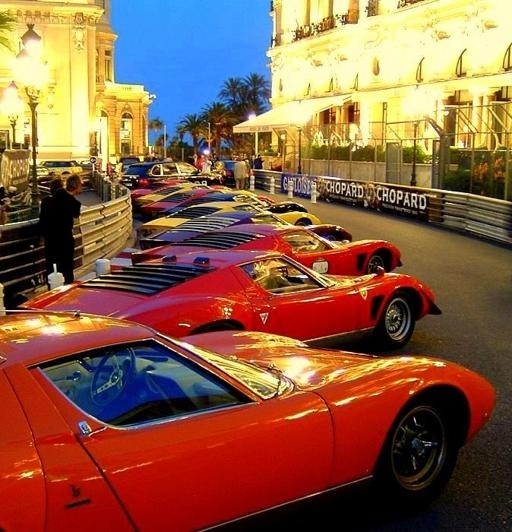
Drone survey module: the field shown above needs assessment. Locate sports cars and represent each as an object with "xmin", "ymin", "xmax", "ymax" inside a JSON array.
[
  {"xmin": 117, "ymin": 155, "xmax": 404, "ymax": 277},
  {"xmin": 0, "ymin": 309, "xmax": 499, "ymax": 530},
  {"xmin": 16, "ymin": 248, "xmax": 442, "ymax": 347}
]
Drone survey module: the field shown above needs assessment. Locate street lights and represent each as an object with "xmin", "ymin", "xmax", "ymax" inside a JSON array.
[
  {"xmin": 400, "ymin": 88, "xmax": 428, "ymax": 188},
  {"xmin": 1, "ymin": 81, "xmax": 24, "ymax": 146},
  {"xmin": 12, "ymin": 20, "xmax": 46, "ymax": 220},
  {"xmin": 287, "ymin": 106, "xmax": 312, "ymax": 174}
]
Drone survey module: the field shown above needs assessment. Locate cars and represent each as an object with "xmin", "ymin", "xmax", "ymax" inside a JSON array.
[{"xmin": 29, "ymin": 159, "xmax": 85, "ymax": 177}]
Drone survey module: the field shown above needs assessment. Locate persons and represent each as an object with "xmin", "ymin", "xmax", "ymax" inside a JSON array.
[
  {"xmin": 196, "ymin": 153, "xmax": 217, "ymax": 175},
  {"xmin": 0, "ymin": 187, "xmax": 10, "ymax": 223},
  {"xmin": 145, "ymin": 152, "xmax": 159, "ymax": 162},
  {"xmin": 254, "ymin": 154, "xmax": 265, "ymax": 169},
  {"xmin": 39, "ymin": 174, "xmax": 84, "ymax": 291},
  {"xmin": 235, "ymin": 159, "xmax": 251, "ymax": 189}
]
